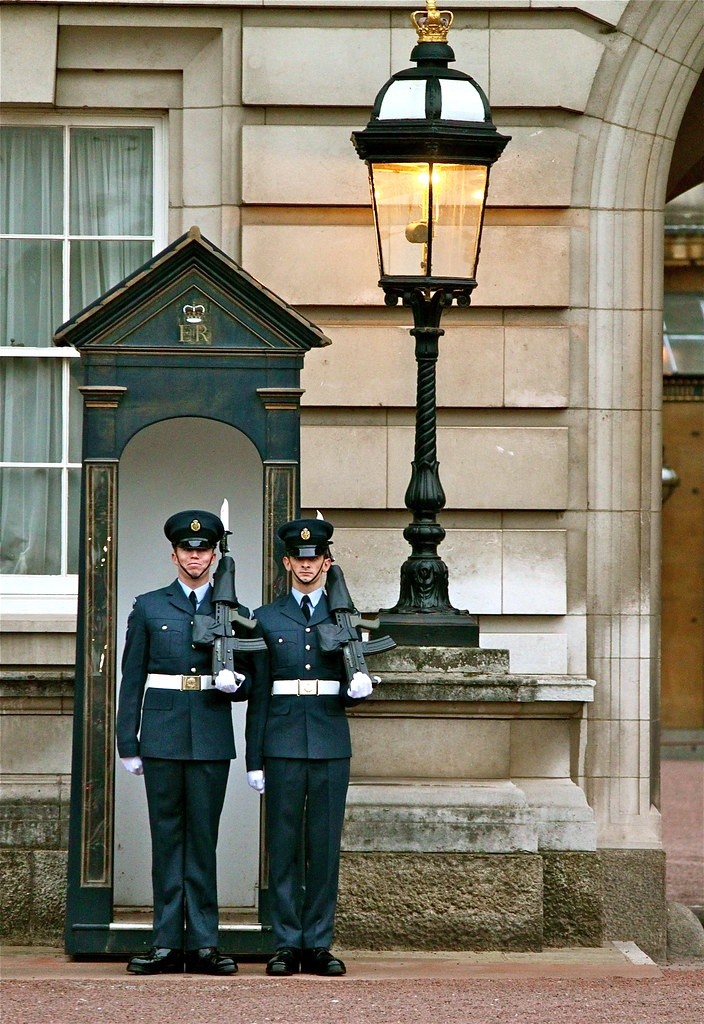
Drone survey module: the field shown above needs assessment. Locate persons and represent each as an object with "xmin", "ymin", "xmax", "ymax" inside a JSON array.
[
  {"xmin": 245, "ymin": 519, "xmax": 375, "ymax": 975},
  {"xmin": 117, "ymin": 505, "xmax": 252, "ymax": 974}
]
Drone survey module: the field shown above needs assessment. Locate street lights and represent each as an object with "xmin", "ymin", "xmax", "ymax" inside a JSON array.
[{"xmin": 356, "ymin": 0, "xmax": 516, "ymax": 651}]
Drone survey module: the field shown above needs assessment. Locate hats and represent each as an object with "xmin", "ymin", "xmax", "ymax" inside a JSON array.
[
  {"xmin": 164, "ymin": 510, "xmax": 225, "ymax": 549},
  {"xmin": 277, "ymin": 519, "xmax": 334, "ymax": 557}
]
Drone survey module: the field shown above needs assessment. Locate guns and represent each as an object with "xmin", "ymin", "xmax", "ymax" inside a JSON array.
[
  {"xmin": 315, "ymin": 509, "xmax": 397, "ymax": 689},
  {"xmin": 211, "ymin": 497, "xmax": 269, "ymax": 685}
]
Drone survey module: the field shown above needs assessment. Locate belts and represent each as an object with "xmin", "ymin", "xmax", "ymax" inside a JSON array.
[
  {"xmin": 271, "ymin": 679, "xmax": 340, "ymax": 696},
  {"xmin": 147, "ymin": 674, "xmax": 216, "ymax": 691}
]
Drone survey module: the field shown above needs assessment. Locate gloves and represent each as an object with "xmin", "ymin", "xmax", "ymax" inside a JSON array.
[
  {"xmin": 347, "ymin": 672, "xmax": 373, "ymax": 699},
  {"xmin": 215, "ymin": 669, "xmax": 245, "ymax": 693},
  {"xmin": 120, "ymin": 756, "xmax": 143, "ymax": 775},
  {"xmin": 247, "ymin": 769, "xmax": 264, "ymax": 793}
]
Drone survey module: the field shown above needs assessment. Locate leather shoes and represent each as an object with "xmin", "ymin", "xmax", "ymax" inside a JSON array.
[
  {"xmin": 185, "ymin": 947, "xmax": 238, "ymax": 974},
  {"xmin": 301, "ymin": 946, "xmax": 346, "ymax": 975},
  {"xmin": 127, "ymin": 946, "xmax": 184, "ymax": 974},
  {"xmin": 266, "ymin": 947, "xmax": 299, "ymax": 975}
]
